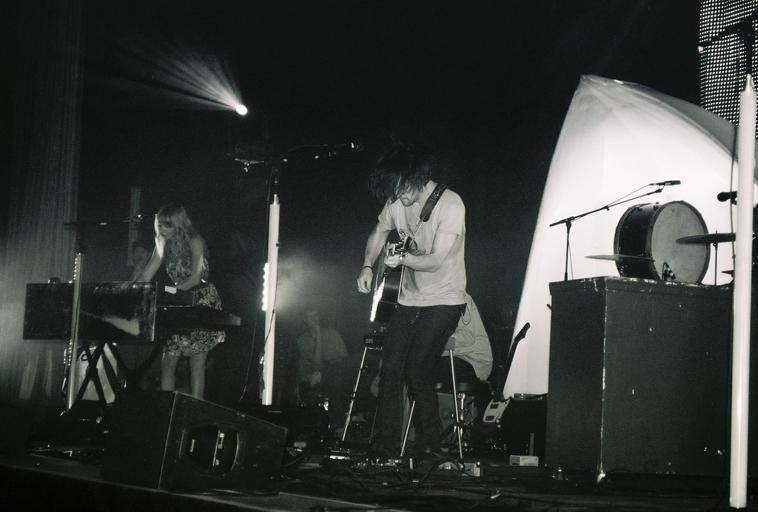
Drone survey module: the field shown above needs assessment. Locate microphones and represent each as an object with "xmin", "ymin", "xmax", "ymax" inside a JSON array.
[
  {"xmin": 346, "ymin": 138, "xmax": 366, "ymax": 152},
  {"xmin": 653, "ymin": 180, "xmax": 681, "ymax": 185},
  {"xmin": 110, "ymin": 213, "xmax": 144, "ymax": 225},
  {"xmin": 717, "ymin": 191, "xmax": 736, "ymax": 202}
]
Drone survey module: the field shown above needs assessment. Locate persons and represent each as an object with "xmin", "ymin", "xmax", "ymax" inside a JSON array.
[
  {"xmin": 123, "ymin": 240, "xmax": 149, "ymax": 282},
  {"xmin": 353, "ymin": 137, "xmax": 469, "ymax": 463},
  {"xmin": 430, "ymin": 289, "xmax": 493, "ymax": 389},
  {"xmin": 294, "ymin": 300, "xmax": 353, "ymax": 447},
  {"xmin": 131, "ymin": 196, "xmax": 225, "ymax": 400}
]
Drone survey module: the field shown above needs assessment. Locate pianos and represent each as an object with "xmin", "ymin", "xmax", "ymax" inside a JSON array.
[{"xmin": 22, "ymin": 281, "xmax": 241, "ymax": 343}]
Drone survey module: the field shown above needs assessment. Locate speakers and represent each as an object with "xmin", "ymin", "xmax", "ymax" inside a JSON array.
[
  {"xmin": 545, "ymin": 275, "xmax": 732, "ymax": 485},
  {"xmin": 103, "ymin": 387, "xmax": 288, "ymax": 492}
]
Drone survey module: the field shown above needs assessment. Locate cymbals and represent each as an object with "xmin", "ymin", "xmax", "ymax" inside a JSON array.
[
  {"xmin": 674, "ymin": 233, "xmax": 735, "ymax": 244},
  {"xmin": 585, "ymin": 255, "xmax": 656, "ymax": 264}
]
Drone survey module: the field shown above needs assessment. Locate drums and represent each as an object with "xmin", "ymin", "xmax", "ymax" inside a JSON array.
[{"xmin": 614, "ymin": 202, "xmax": 710, "ymax": 284}]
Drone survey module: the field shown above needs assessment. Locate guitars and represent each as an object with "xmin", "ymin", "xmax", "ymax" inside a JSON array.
[
  {"xmin": 369, "ymin": 234, "xmax": 414, "ymax": 322},
  {"xmin": 483, "ymin": 323, "xmax": 530, "ymax": 453}
]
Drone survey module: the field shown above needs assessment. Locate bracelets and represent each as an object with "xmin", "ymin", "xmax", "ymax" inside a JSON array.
[{"xmin": 360, "ymin": 265, "xmax": 374, "ymax": 271}]
